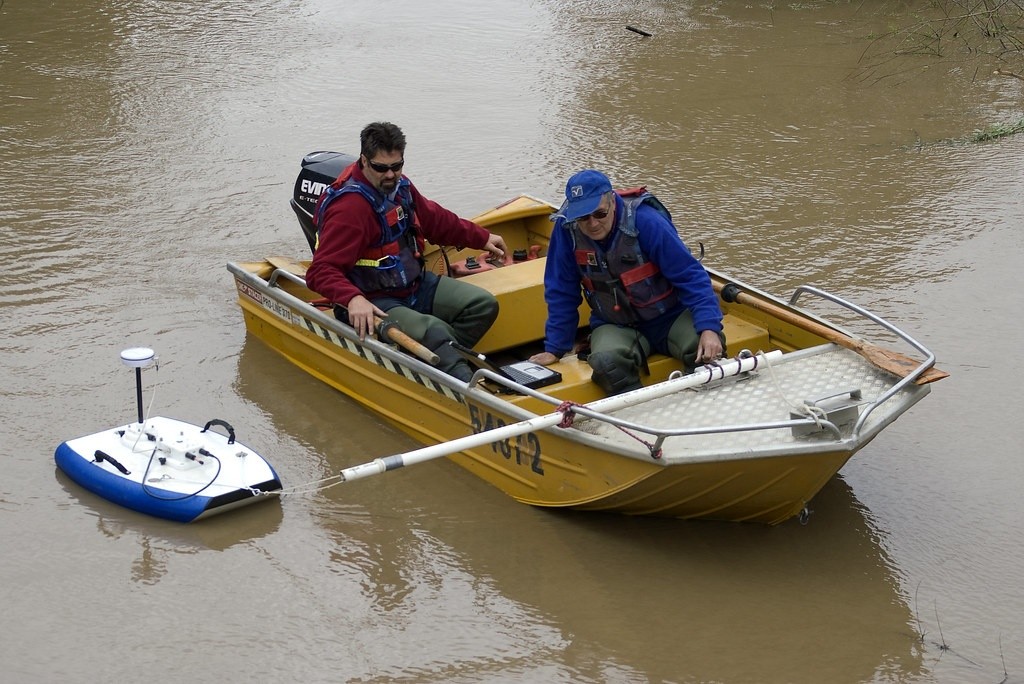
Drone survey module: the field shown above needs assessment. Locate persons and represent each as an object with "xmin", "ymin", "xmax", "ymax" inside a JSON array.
[
  {"xmin": 529, "ymin": 169, "xmax": 729, "ymax": 397},
  {"xmin": 305, "ymin": 122, "xmax": 507, "ymax": 382}
]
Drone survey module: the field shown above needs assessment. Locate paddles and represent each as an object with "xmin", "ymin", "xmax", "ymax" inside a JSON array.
[
  {"xmin": 266, "ymin": 255, "xmax": 441, "ymax": 366},
  {"xmin": 712, "ymin": 277, "xmax": 950, "ymax": 386}
]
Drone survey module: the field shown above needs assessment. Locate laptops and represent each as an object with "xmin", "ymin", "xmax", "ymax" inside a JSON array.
[{"xmin": 448, "ymin": 341, "xmax": 562, "ymax": 395}]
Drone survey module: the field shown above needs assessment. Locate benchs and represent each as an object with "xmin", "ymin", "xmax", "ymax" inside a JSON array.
[
  {"xmin": 467, "ymin": 313, "xmax": 771, "ymax": 416},
  {"xmin": 321, "ymin": 256, "xmax": 593, "ymax": 354}
]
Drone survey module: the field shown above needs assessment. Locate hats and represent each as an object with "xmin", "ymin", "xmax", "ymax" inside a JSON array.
[{"xmin": 566, "ymin": 169, "xmax": 612, "ymax": 221}]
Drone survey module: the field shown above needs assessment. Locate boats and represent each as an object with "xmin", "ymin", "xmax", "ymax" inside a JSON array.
[{"xmin": 222, "ymin": 194, "xmax": 952, "ymax": 525}]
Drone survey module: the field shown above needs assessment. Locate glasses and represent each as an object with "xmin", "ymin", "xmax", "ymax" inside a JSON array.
[
  {"xmin": 367, "ymin": 156, "xmax": 404, "ymax": 173},
  {"xmin": 575, "ymin": 199, "xmax": 610, "ymax": 222}
]
